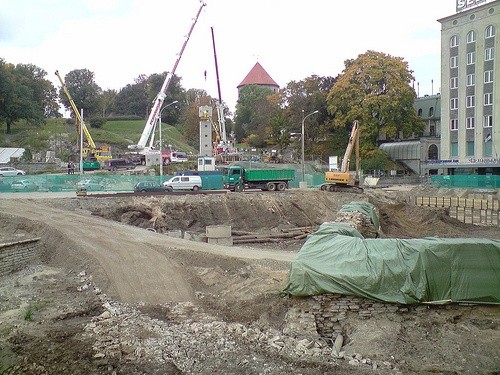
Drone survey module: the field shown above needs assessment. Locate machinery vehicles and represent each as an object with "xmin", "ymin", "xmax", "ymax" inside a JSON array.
[
  {"xmin": 320, "ymin": 117, "xmax": 380, "ymax": 194},
  {"xmin": 117, "ymin": 0, "xmax": 291, "ymax": 166},
  {"xmin": 54, "ymin": 70, "xmax": 112, "ymax": 171}
]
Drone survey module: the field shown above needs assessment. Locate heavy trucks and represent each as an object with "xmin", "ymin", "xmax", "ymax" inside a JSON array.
[{"xmin": 221, "ymin": 165, "xmax": 296, "ymax": 193}]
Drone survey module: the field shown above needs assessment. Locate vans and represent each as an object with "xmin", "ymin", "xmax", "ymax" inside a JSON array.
[{"xmin": 162, "ymin": 175, "xmax": 202, "ymax": 191}]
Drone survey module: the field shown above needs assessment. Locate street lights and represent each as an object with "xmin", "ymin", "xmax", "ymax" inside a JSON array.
[
  {"xmin": 302, "ymin": 109, "xmax": 319, "ymax": 182},
  {"xmin": 159, "ymin": 100, "xmax": 179, "ymax": 177}
]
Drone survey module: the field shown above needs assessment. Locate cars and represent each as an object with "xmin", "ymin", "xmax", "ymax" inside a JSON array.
[
  {"xmin": 0, "ymin": 166, "xmax": 26, "ymax": 177},
  {"xmin": 105, "ymin": 157, "xmax": 137, "ymax": 172},
  {"xmin": 83, "ymin": 156, "xmax": 102, "ymax": 171},
  {"xmin": 133, "ymin": 180, "xmax": 174, "ymax": 193}
]
